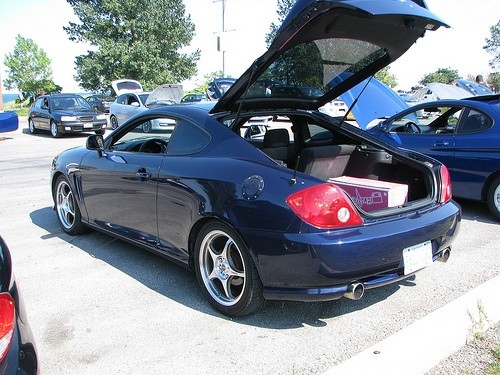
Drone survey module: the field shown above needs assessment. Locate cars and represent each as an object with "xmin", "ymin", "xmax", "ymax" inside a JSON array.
[
  {"xmin": 424, "ymin": 78, "xmax": 496, "ymax": 124},
  {"xmin": 109, "ymin": 79, "xmax": 184, "ymax": 133},
  {"xmin": 27, "ymin": 92, "xmax": 108, "ymax": 138},
  {"xmin": 50, "ymin": 0, "xmax": 465, "ymax": 319},
  {"xmin": 85, "ymin": 94, "xmax": 115, "ymax": 113},
  {"xmin": 329, "ymin": 73, "xmax": 500, "ymax": 222},
  {"xmin": 397, "ymin": 93, "xmax": 429, "ymax": 118},
  {"xmin": 1, "ymin": 235, "xmax": 39, "ymax": 375},
  {"xmin": 181, "ymin": 93, "xmax": 207, "ymax": 102}
]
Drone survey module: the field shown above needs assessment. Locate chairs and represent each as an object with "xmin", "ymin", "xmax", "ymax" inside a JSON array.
[
  {"xmin": 463, "ymin": 114, "xmax": 482, "ymax": 130},
  {"xmin": 262, "ymin": 129, "xmax": 296, "ymax": 169}
]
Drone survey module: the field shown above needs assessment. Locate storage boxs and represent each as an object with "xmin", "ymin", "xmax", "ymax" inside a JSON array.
[{"xmin": 326, "ymin": 176, "xmax": 408, "ymax": 212}]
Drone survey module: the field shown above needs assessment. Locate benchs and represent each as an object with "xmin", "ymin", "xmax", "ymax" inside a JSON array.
[{"xmin": 296, "ymin": 144, "xmax": 366, "ymax": 182}]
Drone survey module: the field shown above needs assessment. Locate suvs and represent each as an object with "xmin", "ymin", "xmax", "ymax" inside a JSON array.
[{"xmin": 268, "ymin": 84, "xmax": 350, "ymax": 120}]
